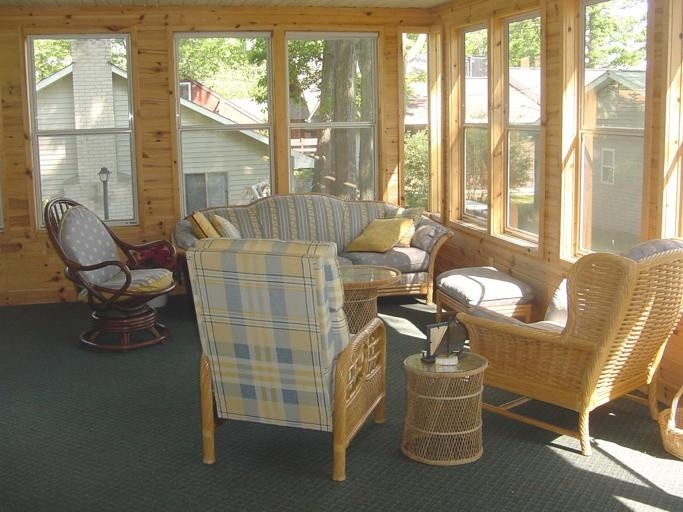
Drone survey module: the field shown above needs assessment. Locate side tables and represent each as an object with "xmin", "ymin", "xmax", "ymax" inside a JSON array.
[{"xmin": 398, "ymin": 350, "xmax": 490, "ymax": 468}]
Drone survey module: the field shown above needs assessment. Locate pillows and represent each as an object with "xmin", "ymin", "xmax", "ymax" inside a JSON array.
[
  {"xmin": 382, "ymin": 205, "xmax": 423, "ymax": 248},
  {"xmin": 187, "ymin": 209, "xmax": 219, "ymax": 241},
  {"xmin": 211, "ymin": 212, "xmax": 244, "ymax": 241},
  {"xmin": 342, "ymin": 215, "xmax": 407, "ymax": 253}
]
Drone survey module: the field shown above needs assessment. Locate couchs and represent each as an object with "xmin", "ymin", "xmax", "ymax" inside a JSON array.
[
  {"xmin": 170, "ymin": 194, "xmax": 455, "ymax": 308},
  {"xmin": 185, "ymin": 234, "xmax": 388, "ymax": 482},
  {"xmin": 454, "ymin": 239, "xmax": 683, "ymax": 457}
]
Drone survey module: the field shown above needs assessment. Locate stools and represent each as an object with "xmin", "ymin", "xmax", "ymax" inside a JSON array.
[{"xmin": 434, "ymin": 265, "xmax": 534, "ymax": 340}]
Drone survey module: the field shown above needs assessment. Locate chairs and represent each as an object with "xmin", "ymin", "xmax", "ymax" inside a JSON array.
[{"xmin": 42, "ymin": 194, "xmax": 180, "ymax": 353}]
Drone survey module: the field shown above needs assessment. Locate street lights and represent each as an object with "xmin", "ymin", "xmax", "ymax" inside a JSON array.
[{"xmin": 97, "ymin": 166, "xmax": 112, "ymax": 221}]
